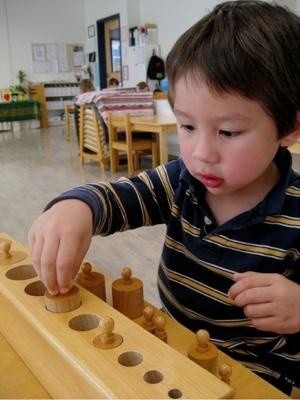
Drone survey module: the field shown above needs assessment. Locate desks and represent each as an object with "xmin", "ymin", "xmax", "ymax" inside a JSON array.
[
  {"xmin": 0, "ymin": 101, "xmax": 41, "ymax": 131},
  {"xmin": 130, "ymin": 116, "xmax": 177, "ymax": 165},
  {"xmin": 76, "ymin": 92, "xmax": 154, "ymax": 172}
]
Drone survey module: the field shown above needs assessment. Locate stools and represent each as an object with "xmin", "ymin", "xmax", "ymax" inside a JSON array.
[
  {"xmin": 0, "ymin": 117, "xmax": 12, "ymax": 132},
  {"xmin": 65, "ymin": 104, "xmax": 77, "ymax": 141}
]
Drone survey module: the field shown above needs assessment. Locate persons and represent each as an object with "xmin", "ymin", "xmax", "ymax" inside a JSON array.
[
  {"xmin": 109, "ymin": 77, "xmax": 120, "ymax": 88},
  {"xmin": 26, "ymin": 0, "xmax": 300, "ymax": 397},
  {"xmin": 77, "ymin": 79, "xmax": 98, "ymax": 105}
]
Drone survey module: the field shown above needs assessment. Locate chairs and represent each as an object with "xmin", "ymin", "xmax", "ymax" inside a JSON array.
[{"xmin": 108, "ymin": 114, "xmax": 158, "ymax": 178}]
